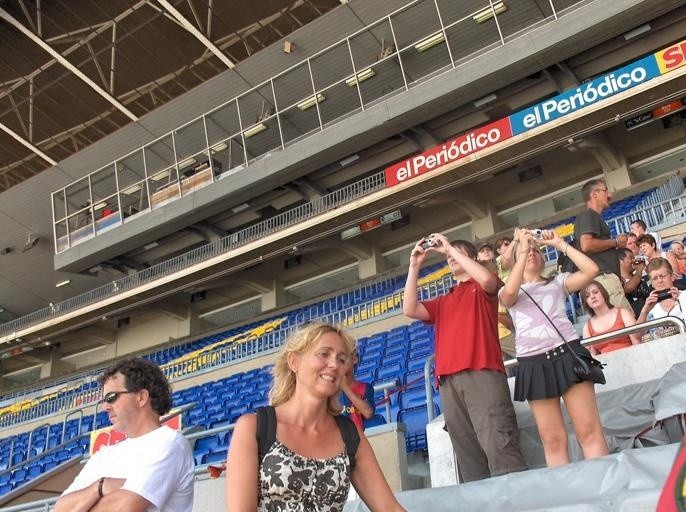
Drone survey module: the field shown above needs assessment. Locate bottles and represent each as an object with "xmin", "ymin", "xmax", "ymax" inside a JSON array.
[{"xmin": 646, "ymin": 313, "xmax": 661, "ymax": 340}]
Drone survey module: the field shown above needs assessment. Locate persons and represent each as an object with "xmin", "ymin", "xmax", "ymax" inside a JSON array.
[
  {"xmin": 403, "ymin": 233, "xmax": 527, "ymax": 484},
  {"xmin": 574, "ymin": 179, "xmax": 637, "ymax": 317},
  {"xmin": 225, "ymin": 321, "xmax": 408, "ymax": 512},
  {"xmin": 51, "ymin": 358, "xmax": 196, "ymax": 511},
  {"xmin": 580, "ymin": 219, "xmax": 686, "ymax": 355},
  {"xmin": 336, "ymin": 348, "xmax": 377, "ymax": 432},
  {"xmin": 497, "ymin": 228, "xmax": 609, "ymax": 467},
  {"xmin": 474, "ymin": 236, "xmax": 518, "ymax": 359}
]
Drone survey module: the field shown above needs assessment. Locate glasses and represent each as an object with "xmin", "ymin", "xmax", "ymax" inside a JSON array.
[{"xmin": 102, "ymin": 389, "xmax": 138, "ymax": 403}]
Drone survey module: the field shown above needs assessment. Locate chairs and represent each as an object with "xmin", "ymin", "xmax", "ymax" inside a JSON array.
[{"xmin": 0, "ymin": 184, "xmax": 662, "ymax": 510}]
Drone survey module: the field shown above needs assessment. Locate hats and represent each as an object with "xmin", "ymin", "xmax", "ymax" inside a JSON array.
[{"xmin": 476, "ymin": 241, "xmax": 493, "ymax": 252}]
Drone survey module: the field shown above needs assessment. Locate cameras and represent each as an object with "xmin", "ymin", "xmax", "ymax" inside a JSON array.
[
  {"xmin": 633, "ymin": 256, "xmax": 647, "ymax": 265},
  {"xmin": 420, "ymin": 235, "xmax": 438, "ymax": 249},
  {"xmin": 653, "ymin": 288, "xmax": 672, "ymax": 302},
  {"xmin": 529, "ymin": 228, "xmax": 545, "ymax": 240}
]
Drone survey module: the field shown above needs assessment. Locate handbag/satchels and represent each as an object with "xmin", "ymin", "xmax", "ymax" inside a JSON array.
[
  {"xmin": 557, "ymin": 240, "xmax": 581, "ymax": 272},
  {"xmin": 574, "ymin": 351, "xmax": 605, "ymax": 384},
  {"xmin": 641, "ymin": 328, "xmax": 676, "ymax": 343}
]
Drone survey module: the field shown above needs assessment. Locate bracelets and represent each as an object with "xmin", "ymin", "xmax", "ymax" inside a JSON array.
[{"xmin": 99, "ymin": 477, "xmax": 105, "ymax": 500}]
